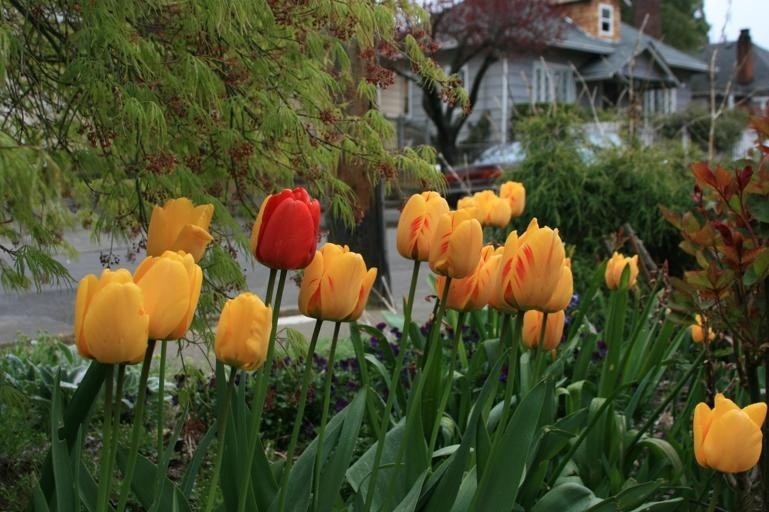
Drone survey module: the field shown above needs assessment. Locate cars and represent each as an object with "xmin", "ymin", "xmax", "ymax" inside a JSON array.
[{"xmin": 444, "ymin": 140, "xmax": 525, "ymax": 209}]
